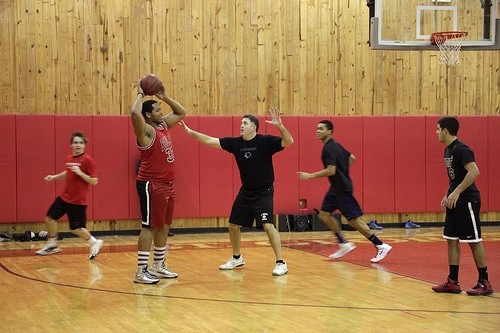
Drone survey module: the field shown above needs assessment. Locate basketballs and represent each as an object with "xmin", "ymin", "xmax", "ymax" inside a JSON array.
[{"xmin": 140, "ymin": 74, "xmax": 163, "ymax": 95}]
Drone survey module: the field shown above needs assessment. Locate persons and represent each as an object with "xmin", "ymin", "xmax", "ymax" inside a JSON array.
[
  {"xmin": 296, "ymin": 120, "xmax": 392, "ymax": 263},
  {"xmin": 431, "ymin": 117, "xmax": 494, "ymax": 296},
  {"xmin": 131, "ymin": 78, "xmax": 186, "ymax": 283},
  {"xmin": 36, "ymin": 131, "xmax": 104, "ymax": 260},
  {"xmin": 177, "ymin": 107, "xmax": 294, "ymax": 275}
]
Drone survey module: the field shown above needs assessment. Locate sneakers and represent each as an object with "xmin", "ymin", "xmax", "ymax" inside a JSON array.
[
  {"xmin": 148, "ymin": 261, "xmax": 179, "ymax": 278},
  {"xmin": 432, "ymin": 276, "xmax": 462, "ymax": 293},
  {"xmin": 219, "ymin": 254, "xmax": 245, "ymax": 270},
  {"xmin": 466, "ymin": 280, "xmax": 494, "ymax": 296},
  {"xmin": 36, "ymin": 242, "xmax": 61, "ymax": 255},
  {"xmin": 369, "ymin": 220, "xmax": 383, "ymax": 230},
  {"xmin": 89, "ymin": 239, "xmax": 104, "ymax": 259},
  {"xmin": 328, "ymin": 241, "xmax": 357, "ymax": 260},
  {"xmin": 370, "ymin": 243, "xmax": 392, "ymax": 263},
  {"xmin": 405, "ymin": 220, "xmax": 421, "ymax": 229},
  {"xmin": 134, "ymin": 265, "xmax": 160, "ymax": 284},
  {"xmin": 272, "ymin": 260, "xmax": 288, "ymax": 276}
]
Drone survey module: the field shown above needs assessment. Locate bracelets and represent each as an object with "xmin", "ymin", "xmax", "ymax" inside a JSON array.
[{"xmin": 137, "ymin": 92, "xmax": 143, "ymax": 98}]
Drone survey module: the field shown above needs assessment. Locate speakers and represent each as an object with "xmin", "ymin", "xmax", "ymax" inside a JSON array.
[
  {"xmin": 277, "ymin": 214, "xmax": 311, "ymax": 233},
  {"xmin": 308, "ymin": 213, "xmax": 343, "ymax": 231}
]
